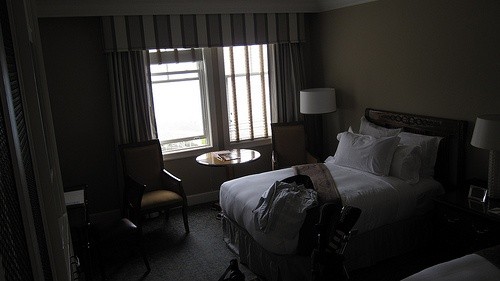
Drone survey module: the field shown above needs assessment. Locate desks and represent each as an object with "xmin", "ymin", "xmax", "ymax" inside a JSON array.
[
  {"xmin": 62, "ymin": 176, "xmax": 93, "ymax": 279},
  {"xmin": 196, "ymin": 148, "xmax": 261, "ymax": 211}
]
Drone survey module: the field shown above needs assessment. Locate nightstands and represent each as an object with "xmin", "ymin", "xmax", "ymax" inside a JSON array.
[{"xmin": 439, "ymin": 190, "xmax": 500, "ymax": 253}]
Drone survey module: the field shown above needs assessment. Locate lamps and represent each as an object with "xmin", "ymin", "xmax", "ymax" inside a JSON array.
[
  {"xmin": 299, "ymin": 88, "xmax": 336, "ymax": 162},
  {"xmin": 471, "ymin": 115, "xmax": 500, "ymax": 199}
]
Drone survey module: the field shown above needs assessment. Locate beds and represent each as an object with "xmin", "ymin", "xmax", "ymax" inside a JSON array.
[
  {"xmin": 400, "ymin": 244, "xmax": 500, "ymax": 281},
  {"xmin": 217, "ymin": 108, "xmax": 467, "ymax": 281}
]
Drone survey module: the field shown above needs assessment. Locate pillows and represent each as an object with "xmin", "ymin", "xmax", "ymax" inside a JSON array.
[{"xmin": 324, "ymin": 115, "xmax": 445, "ymax": 181}]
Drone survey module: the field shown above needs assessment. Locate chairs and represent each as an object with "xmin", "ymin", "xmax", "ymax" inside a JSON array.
[
  {"xmin": 86, "ymin": 175, "xmax": 152, "ymax": 281},
  {"xmin": 270, "ymin": 122, "xmax": 318, "ymax": 170},
  {"xmin": 117, "ymin": 140, "xmax": 190, "ymax": 237}
]
